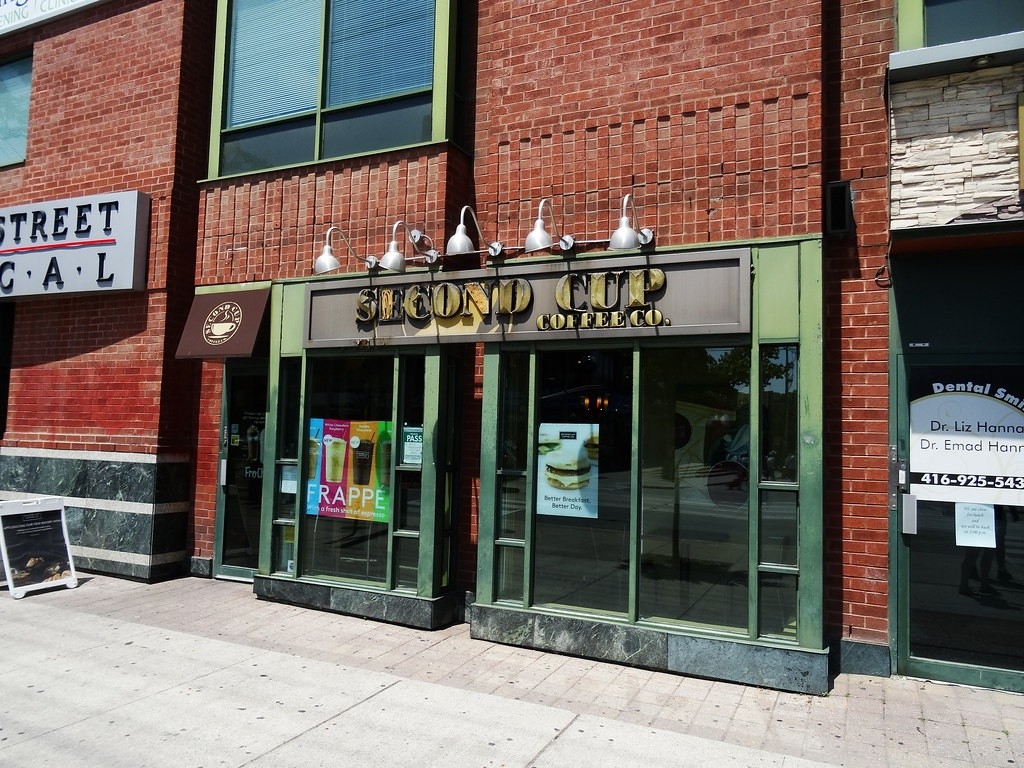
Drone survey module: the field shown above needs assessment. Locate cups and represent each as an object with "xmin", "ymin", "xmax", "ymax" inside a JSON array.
[
  {"xmin": 326, "ymin": 442, "xmax": 346, "ymax": 482},
  {"xmin": 353, "ymin": 443, "xmax": 375, "ymax": 485},
  {"xmin": 380, "ymin": 444, "xmax": 391, "ymax": 485},
  {"xmin": 247, "ymin": 434, "xmax": 258, "ymax": 461},
  {"xmin": 260, "ymin": 435, "xmax": 264, "ymax": 463},
  {"xmin": 308, "ymin": 439, "xmax": 320, "ymax": 479}
]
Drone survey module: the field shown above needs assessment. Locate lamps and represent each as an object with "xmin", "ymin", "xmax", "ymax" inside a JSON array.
[
  {"xmin": 524, "ymin": 198, "xmax": 575, "ymax": 254},
  {"xmin": 446, "ymin": 206, "xmax": 504, "ymax": 257},
  {"xmin": 379, "ymin": 221, "xmax": 439, "ymax": 272},
  {"xmin": 315, "ymin": 227, "xmax": 379, "ymax": 276},
  {"xmin": 608, "ymin": 194, "xmax": 653, "ymax": 252}
]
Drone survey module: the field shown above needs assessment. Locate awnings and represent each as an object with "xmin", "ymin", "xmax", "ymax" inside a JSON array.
[{"xmin": 175, "ymin": 286, "xmax": 272, "ymax": 359}]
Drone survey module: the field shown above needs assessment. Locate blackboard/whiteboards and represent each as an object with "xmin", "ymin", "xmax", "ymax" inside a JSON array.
[{"xmin": 0, "ymin": 496, "xmax": 78, "ymax": 599}]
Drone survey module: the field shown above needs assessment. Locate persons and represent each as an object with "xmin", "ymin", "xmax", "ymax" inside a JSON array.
[{"xmin": 714, "ymin": 443, "xmax": 797, "ymax": 492}]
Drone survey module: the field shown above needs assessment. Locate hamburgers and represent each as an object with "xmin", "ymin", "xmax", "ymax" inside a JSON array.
[
  {"xmin": 545, "ymin": 451, "xmax": 591, "ymax": 489},
  {"xmin": 584, "ymin": 435, "xmax": 599, "ymax": 459}
]
